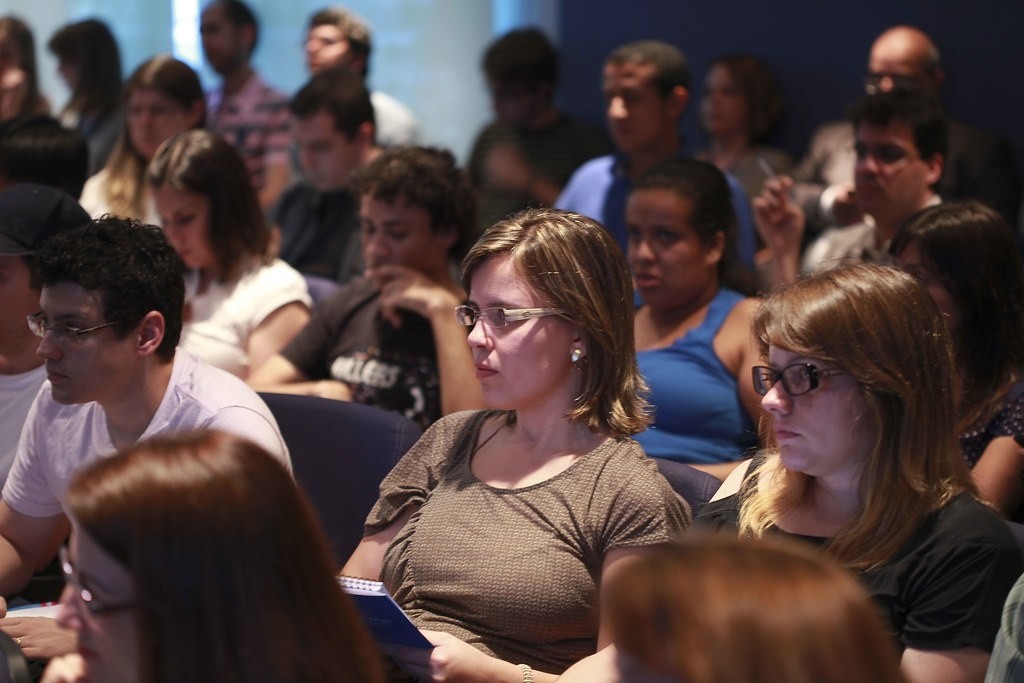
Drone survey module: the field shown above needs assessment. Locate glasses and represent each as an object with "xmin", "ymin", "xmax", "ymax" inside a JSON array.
[
  {"xmin": 27, "ymin": 310, "xmax": 146, "ymax": 344},
  {"xmin": 751, "ymin": 361, "xmax": 851, "ymax": 395},
  {"xmin": 453, "ymin": 304, "xmax": 572, "ymax": 326},
  {"xmin": 58, "ymin": 544, "xmax": 142, "ymax": 616}
]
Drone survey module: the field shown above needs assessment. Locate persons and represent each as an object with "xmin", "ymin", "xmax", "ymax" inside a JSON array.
[
  {"xmin": 242, "ymin": 145, "xmax": 485, "ymax": 431},
  {"xmin": 199, "ymin": 0, "xmax": 294, "ymax": 214},
  {"xmin": 0, "ymin": 14, "xmax": 48, "ymax": 125},
  {"xmin": 797, "ymin": 24, "xmax": 1008, "ymax": 209},
  {"xmin": 0, "ymin": 180, "xmax": 385, "ymax": 683},
  {"xmin": 552, "ymin": 258, "xmax": 1022, "ymax": 683},
  {"xmin": 602, "ymin": 529, "xmax": 909, "ymax": 683},
  {"xmin": 552, "ymin": 41, "xmax": 759, "ymax": 310},
  {"xmin": 335, "ymin": 205, "xmax": 691, "ymax": 683},
  {"xmin": 269, "ymin": 67, "xmax": 388, "ymax": 283},
  {"xmin": 78, "ymin": 54, "xmax": 206, "ymax": 227},
  {"xmin": 887, "ymin": 202, "xmax": 1024, "ymax": 514},
  {"xmin": 469, "ymin": 27, "xmax": 607, "ymax": 237},
  {"xmin": 303, "ymin": 6, "xmax": 419, "ymax": 148},
  {"xmin": 753, "ymin": 90, "xmax": 949, "ymax": 295},
  {"xmin": 46, "ymin": 18, "xmax": 125, "ymax": 178},
  {"xmin": 150, "ymin": 128, "xmax": 312, "ymax": 381},
  {"xmin": 690, "ymin": 54, "xmax": 794, "ymax": 232},
  {"xmin": 623, "ymin": 156, "xmax": 772, "ymax": 522}
]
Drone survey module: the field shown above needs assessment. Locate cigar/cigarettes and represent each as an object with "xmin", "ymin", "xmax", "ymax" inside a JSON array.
[{"xmin": 759, "ymin": 160, "xmax": 776, "ymax": 179}]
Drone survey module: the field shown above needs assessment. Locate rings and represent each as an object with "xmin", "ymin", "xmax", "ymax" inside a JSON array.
[{"xmin": 17, "ymin": 638, "xmax": 22, "ymax": 648}]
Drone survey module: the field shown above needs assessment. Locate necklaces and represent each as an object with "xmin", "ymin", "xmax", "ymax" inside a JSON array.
[{"xmin": 473, "ymin": 420, "xmax": 507, "ymax": 458}]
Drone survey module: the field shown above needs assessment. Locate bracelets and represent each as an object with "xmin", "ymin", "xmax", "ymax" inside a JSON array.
[{"xmin": 518, "ymin": 663, "xmax": 534, "ymax": 683}]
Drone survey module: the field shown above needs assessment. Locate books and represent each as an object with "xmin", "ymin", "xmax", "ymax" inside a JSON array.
[{"xmin": 339, "ymin": 573, "xmax": 433, "ymax": 648}]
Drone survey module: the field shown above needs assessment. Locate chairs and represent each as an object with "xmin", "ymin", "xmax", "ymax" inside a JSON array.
[
  {"xmin": 259, "ymin": 391, "xmax": 422, "ymax": 575},
  {"xmin": 645, "ymin": 455, "xmax": 723, "ymax": 522}
]
